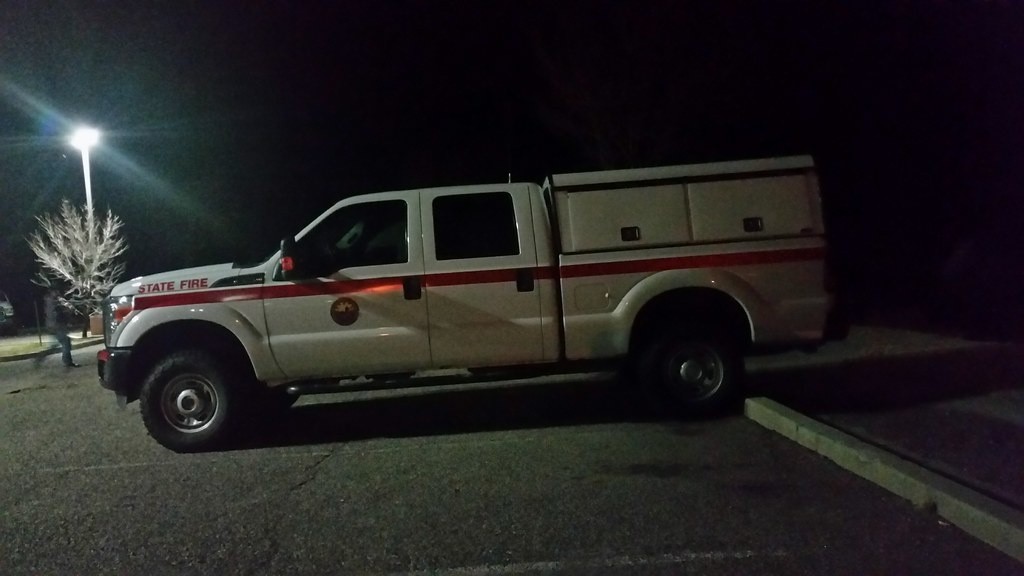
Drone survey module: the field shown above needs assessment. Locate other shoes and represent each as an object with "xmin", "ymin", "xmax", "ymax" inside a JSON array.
[{"xmin": 66, "ymin": 363, "xmax": 78, "ymax": 368}]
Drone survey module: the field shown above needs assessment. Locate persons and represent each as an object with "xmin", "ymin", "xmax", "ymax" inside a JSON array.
[{"xmin": 33, "ymin": 291, "xmax": 83, "ymax": 369}]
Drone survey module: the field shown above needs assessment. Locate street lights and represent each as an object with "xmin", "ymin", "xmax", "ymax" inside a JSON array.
[{"xmin": 69, "ymin": 126, "xmax": 101, "ymax": 244}]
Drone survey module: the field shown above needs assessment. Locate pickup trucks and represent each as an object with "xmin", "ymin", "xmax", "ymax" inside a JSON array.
[{"xmin": 96, "ymin": 154, "xmax": 830, "ymax": 450}]
